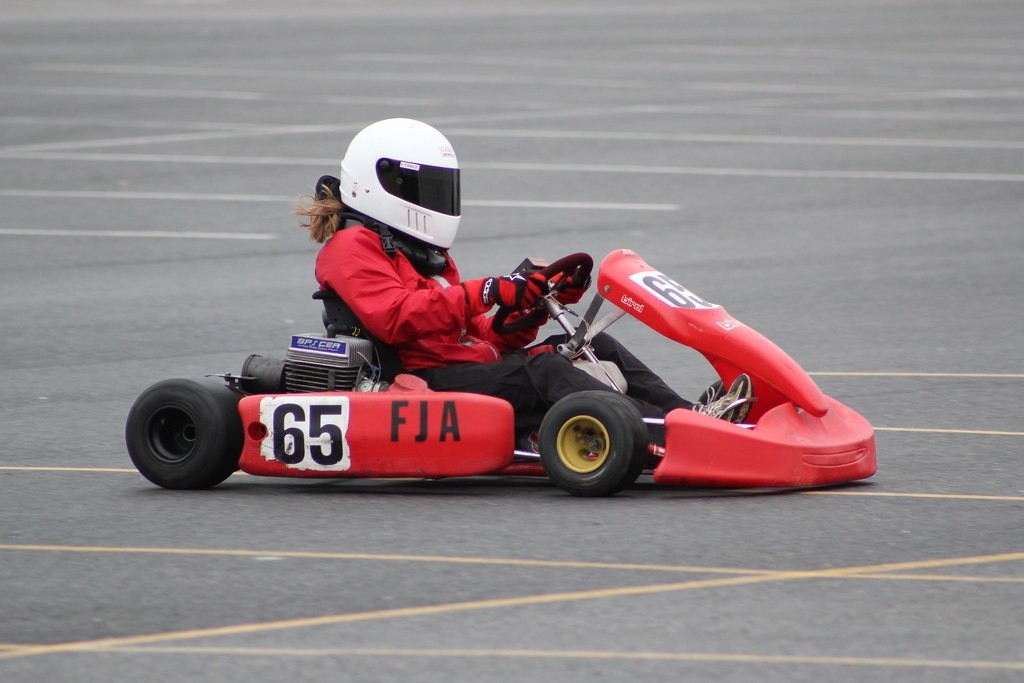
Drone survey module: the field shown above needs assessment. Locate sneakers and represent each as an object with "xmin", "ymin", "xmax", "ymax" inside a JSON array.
[{"xmin": 691, "ymin": 372, "xmax": 754, "ymax": 424}]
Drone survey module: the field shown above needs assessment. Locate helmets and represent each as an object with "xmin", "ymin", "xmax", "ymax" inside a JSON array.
[{"xmin": 339, "ymin": 117, "xmax": 462, "ymax": 249}]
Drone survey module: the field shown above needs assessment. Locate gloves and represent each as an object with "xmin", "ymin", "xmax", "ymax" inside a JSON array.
[
  {"xmin": 482, "ymin": 272, "xmax": 546, "ymax": 309},
  {"xmin": 546, "ymin": 271, "xmax": 592, "ymax": 304}
]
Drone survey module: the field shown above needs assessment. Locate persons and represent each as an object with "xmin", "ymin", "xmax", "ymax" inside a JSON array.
[{"xmin": 296, "ymin": 119, "xmax": 754, "ymax": 461}]
